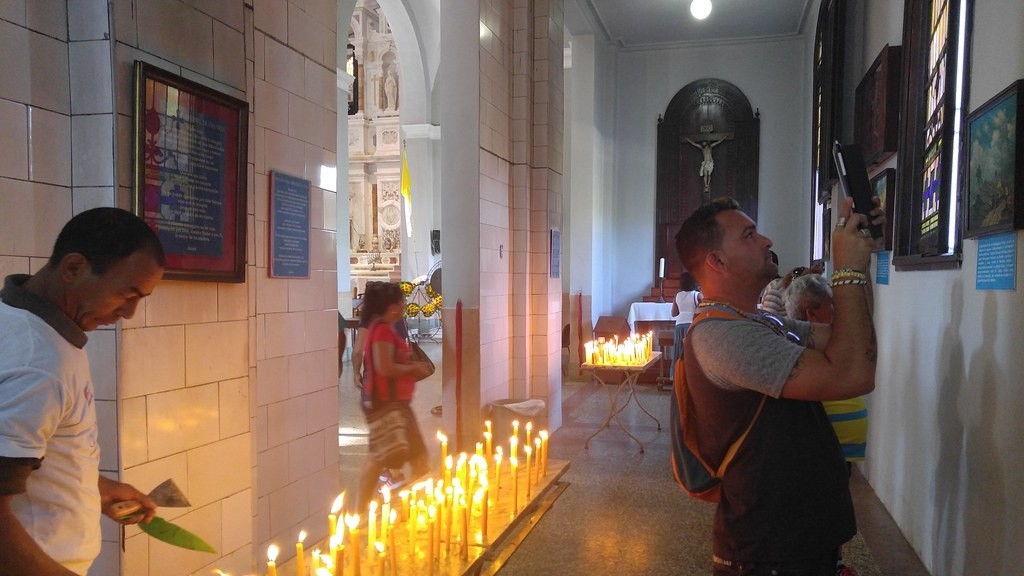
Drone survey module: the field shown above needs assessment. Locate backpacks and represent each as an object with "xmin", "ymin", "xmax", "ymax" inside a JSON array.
[{"xmin": 668, "ymin": 310, "xmax": 772, "ymax": 503}]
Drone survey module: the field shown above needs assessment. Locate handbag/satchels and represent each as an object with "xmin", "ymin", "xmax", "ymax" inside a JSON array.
[
  {"xmin": 365, "ymin": 401, "xmax": 411, "ymax": 469},
  {"xmin": 406, "ymin": 339, "xmax": 436, "ymax": 383}
]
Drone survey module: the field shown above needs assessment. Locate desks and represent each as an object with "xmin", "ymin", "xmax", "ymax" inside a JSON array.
[
  {"xmin": 627, "ymin": 302, "xmax": 762, "ymax": 384},
  {"xmin": 581, "ymin": 351, "xmax": 663, "ymax": 453},
  {"xmin": 655, "ymin": 330, "xmax": 674, "ymax": 393}
]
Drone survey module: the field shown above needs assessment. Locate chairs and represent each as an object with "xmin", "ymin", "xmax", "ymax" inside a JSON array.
[{"xmin": 562, "ymin": 323, "xmax": 570, "ymax": 377}]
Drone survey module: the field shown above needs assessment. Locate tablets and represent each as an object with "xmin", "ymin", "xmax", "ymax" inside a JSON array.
[{"xmin": 833, "ymin": 144, "xmax": 883, "ymax": 238}]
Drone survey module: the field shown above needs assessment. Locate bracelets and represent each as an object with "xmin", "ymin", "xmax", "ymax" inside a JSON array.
[{"xmin": 828, "ymin": 268, "xmax": 867, "ymax": 288}]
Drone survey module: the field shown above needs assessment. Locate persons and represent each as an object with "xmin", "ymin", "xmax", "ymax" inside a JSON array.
[
  {"xmin": 338, "ymin": 281, "xmax": 437, "ymax": 514},
  {"xmin": 384, "ymin": 71, "xmax": 397, "ymax": 109},
  {"xmin": 668, "ymin": 193, "xmax": 878, "ymax": 576},
  {"xmin": 685, "ymin": 135, "xmax": 728, "ymax": 188},
  {"xmin": 0, "ymin": 206, "xmax": 170, "ymax": 576}
]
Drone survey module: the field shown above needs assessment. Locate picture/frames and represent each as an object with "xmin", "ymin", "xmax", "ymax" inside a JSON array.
[
  {"xmin": 870, "ymin": 168, "xmax": 894, "ymax": 253},
  {"xmin": 853, "ymin": 42, "xmax": 901, "ymax": 172},
  {"xmin": 961, "ymin": 79, "xmax": 1024, "ymax": 239}
]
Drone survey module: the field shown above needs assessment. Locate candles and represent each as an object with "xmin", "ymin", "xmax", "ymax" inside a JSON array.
[
  {"xmin": 214, "ymin": 419, "xmax": 548, "ymax": 576},
  {"xmin": 659, "ymin": 258, "xmax": 665, "ymax": 277},
  {"xmin": 585, "ymin": 330, "xmax": 653, "ymax": 366}
]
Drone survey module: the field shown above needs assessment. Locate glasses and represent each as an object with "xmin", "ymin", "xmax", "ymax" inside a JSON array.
[{"xmin": 790, "ymin": 266, "xmax": 805, "ymax": 284}]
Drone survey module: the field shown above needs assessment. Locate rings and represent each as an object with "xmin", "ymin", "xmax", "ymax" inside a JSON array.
[
  {"xmin": 835, "ymin": 216, "xmax": 846, "ymax": 226},
  {"xmin": 860, "ymin": 228, "xmax": 871, "ymax": 238}
]
